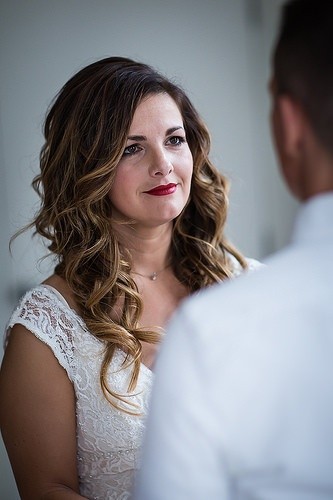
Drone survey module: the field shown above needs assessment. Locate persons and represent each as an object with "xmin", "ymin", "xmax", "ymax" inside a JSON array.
[
  {"xmin": 129, "ymin": 2, "xmax": 333, "ymax": 500},
  {"xmin": 1, "ymin": 57, "xmax": 267, "ymax": 500}
]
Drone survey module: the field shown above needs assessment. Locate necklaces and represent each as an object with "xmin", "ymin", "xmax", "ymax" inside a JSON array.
[{"xmin": 130, "ymin": 263, "xmax": 172, "ymax": 281}]
